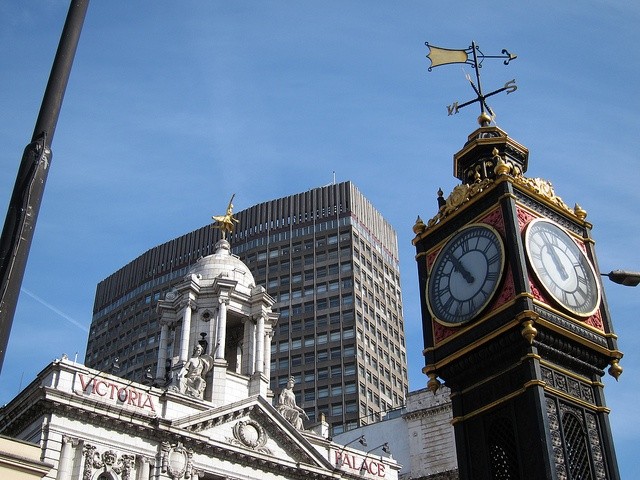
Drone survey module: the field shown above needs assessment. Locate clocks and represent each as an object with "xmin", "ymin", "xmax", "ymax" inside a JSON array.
[
  {"xmin": 425, "ymin": 221, "xmax": 505, "ymax": 328},
  {"xmin": 523, "ymin": 217, "xmax": 601, "ymax": 318}
]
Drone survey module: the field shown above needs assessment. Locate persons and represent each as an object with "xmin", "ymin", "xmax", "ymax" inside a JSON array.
[
  {"xmin": 274, "ymin": 375, "xmax": 310, "ymax": 428},
  {"xmin": 210, "ymin": 203, "xmax": 240, "ymax": 239},
  {"xmin": 177, "ymin": 339, "xmax": 225, "ymax": 397}
]
